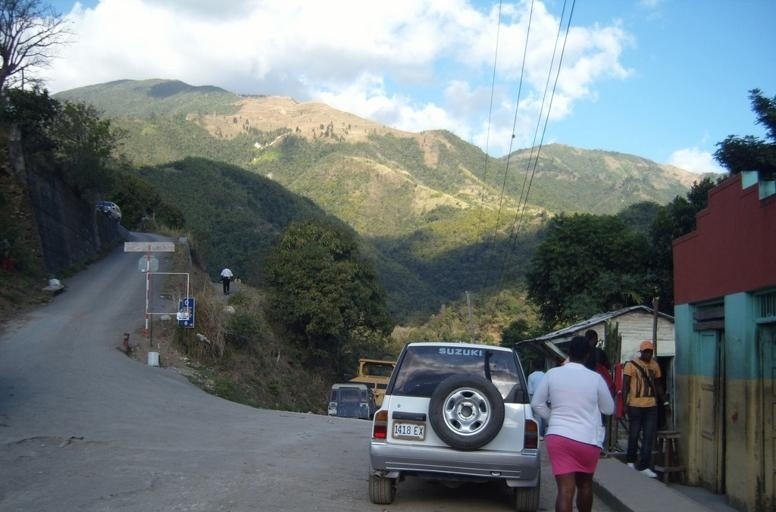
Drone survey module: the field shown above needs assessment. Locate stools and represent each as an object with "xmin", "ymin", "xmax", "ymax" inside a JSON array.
[{"xmin": 653, "ymin": 427, "xmax": 688, "ymax": 486}]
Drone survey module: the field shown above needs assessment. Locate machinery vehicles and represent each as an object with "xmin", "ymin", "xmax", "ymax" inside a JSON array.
[{"xmin": 347, "ymin": 358, "xmax": 399, "ymax": 408}]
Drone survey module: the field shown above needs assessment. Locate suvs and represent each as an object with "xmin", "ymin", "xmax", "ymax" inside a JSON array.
[{"xmin": 367, "ymin": 339, "xmax": 544, "ymax": 512}]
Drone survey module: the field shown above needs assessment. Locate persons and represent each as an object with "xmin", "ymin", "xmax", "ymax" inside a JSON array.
[
  {"xmin": 582, "ymin": 346, "xmax": 615, "ymax": 398},
  {"xmin": 219, "ymin": 264, "xmax": 233, "ymax": 295},
  {"xmin": 623, "ymin": 339, "xmax": 668, "ymax": 478},
  {"xmin": 529, "ymin": 334, "xmax": 618, "ymax": 511},
  {"xmin": 527, "ymin": 355, "xmax": 548, "ymax": 440},
  {"xmin": 585, "ymin": 329, "xmax": 612, "ymax": 456}
]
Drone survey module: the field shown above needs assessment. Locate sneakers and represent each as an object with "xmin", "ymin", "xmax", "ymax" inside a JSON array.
[
  {"xmin": 640, "ymin": 468, "xmax": 658, "ymax": 478},
  {"xmin": 627, "ymin": 462, "xmax": 634, "ymax": 469}
]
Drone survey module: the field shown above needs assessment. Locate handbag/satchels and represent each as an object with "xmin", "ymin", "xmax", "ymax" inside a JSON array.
[{"xmin": 656, "ymin": 400, "xmax": 668, "ymax": 431}]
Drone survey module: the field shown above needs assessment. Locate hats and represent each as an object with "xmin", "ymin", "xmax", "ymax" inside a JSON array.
[{"xmin": 639, "ymin": 340, "xmax": 655, "ymax": 351}]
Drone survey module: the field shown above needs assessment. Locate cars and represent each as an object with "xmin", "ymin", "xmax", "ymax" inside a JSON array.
[{"xmin": 94, "ymin": 199, "xmax": 122, "ymax": 225}]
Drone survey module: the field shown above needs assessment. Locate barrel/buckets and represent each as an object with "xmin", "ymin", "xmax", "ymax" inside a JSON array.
[{"xmin": 147, "ymin": 351, "xmax": 160, "ymax": 366}]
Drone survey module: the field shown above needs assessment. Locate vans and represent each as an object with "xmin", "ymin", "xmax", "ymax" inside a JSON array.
[{"xmin": 328, "ymin": 381, "xmax": 378, "ymax": 419}]
